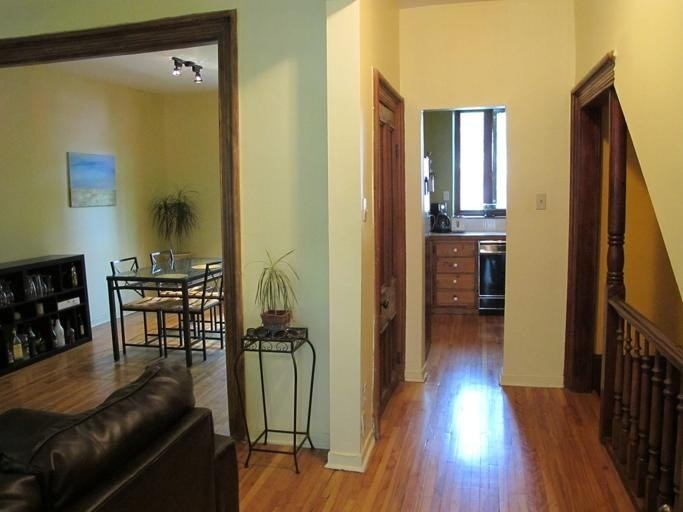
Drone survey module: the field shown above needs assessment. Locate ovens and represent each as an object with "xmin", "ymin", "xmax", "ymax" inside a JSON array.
[{"xmin": 476, "ymin": 240, "xmax": 505, "ymax": 310}]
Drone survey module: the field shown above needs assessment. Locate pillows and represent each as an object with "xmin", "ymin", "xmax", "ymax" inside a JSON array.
[{"xmin": 26, "ymin": 360, "xmax": 195, "ymax": 510}]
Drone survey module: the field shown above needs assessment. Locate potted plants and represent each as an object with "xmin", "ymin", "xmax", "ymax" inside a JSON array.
[
  {"xmin": 146, "ymin": 182, "xmax": 200, "ymax": 270},
  {"xmin": 253, "ymin": 248, "xmax": 302, "ymax": 331}
]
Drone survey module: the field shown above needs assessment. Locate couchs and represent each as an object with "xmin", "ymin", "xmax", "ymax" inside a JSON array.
[{"xmin": 0, "ymin": 359, "xmax": 240, "ymax": 511}]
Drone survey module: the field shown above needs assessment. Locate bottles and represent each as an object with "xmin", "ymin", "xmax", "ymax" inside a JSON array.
[{"xmin": 0, "ymin": 261, "xmax": 87, "ymax": 360}]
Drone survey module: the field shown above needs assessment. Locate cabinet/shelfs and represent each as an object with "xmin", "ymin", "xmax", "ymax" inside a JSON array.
[
  {"xmin": 426, "ymin": 235, "xmax": 478, "ymax": 315},
  {"xmin": 0, "ymin": 254, "xmax": 93, "ymax": 377}
]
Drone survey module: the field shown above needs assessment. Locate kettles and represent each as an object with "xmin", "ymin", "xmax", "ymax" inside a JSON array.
[{"xmin": 451, "ymin": 216, "xmax": 465, "ymax": 232}]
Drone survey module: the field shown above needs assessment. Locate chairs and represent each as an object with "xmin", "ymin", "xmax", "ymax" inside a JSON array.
[{"xmin": 106, "ymin": 248, "xmax": 224, "ymax": 367}]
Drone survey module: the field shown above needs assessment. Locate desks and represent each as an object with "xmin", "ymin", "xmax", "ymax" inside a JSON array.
[{"xmin": 233, "ymin": 327, "xmax": 316, "ymax": 474}]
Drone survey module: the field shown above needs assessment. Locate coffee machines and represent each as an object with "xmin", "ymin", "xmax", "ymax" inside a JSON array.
[{"xmin": 431, "ymin": 202, "xmax": 451, "ymax": 233}]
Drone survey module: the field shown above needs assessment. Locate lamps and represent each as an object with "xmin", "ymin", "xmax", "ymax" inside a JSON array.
[{"xmin": 171, "ymin": 56, "xmax": 204, "ymax": 84}]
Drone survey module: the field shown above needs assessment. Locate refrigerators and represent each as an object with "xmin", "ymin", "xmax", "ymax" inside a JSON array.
[{"xmin": 423, "ymin": 154, "xmax": 432, "ymax": 350}]
